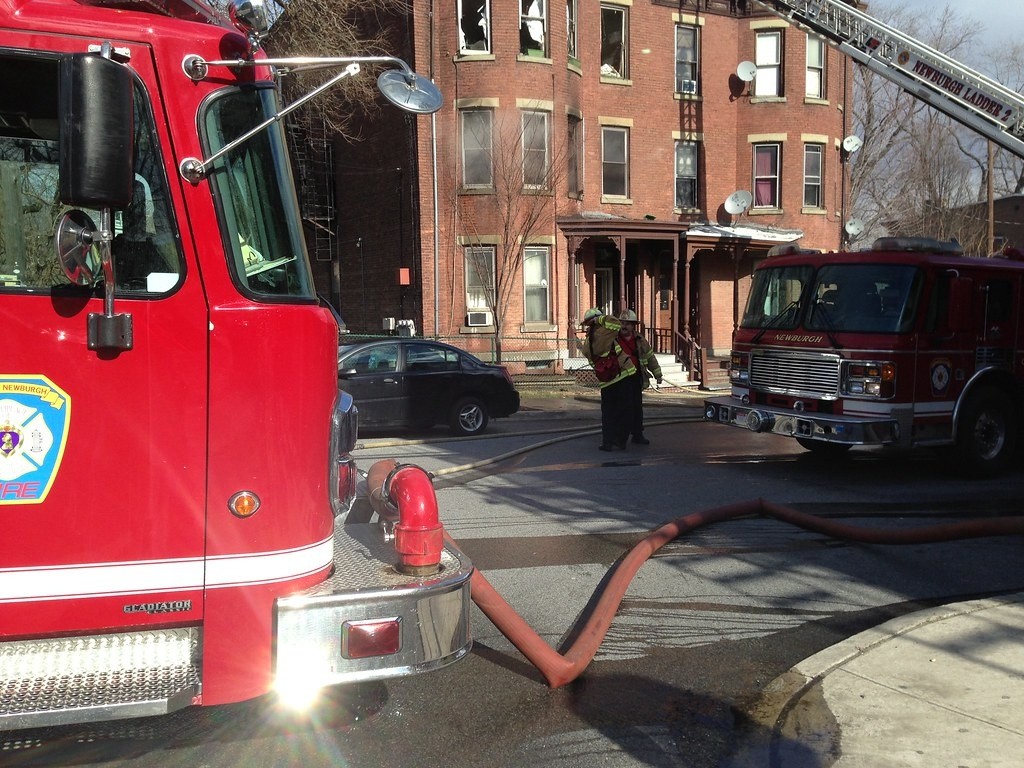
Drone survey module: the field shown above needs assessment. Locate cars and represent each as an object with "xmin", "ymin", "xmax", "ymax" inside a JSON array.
[{"xmin": 338, "ymin": 340, "xmax": 523, "ymax": 436}]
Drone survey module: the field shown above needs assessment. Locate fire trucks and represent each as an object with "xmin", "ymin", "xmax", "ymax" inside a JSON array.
[
  {"xmin": 1, "ymin": 0, "xmax": 475, "ymax": 730},
  {"xmin": 704, "ymin": 236, "xmax": 1024, "ymax": 481}
]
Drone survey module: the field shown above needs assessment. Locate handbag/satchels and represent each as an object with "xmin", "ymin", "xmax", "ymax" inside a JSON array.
[{"xmin": 590, "ymin": 328, "xmax": 621, "ymax": 382}]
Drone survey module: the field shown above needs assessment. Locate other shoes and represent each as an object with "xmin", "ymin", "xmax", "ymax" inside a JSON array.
[
  {"xmin": 598, "ymin": 442, "xmax": 613, "ymax": 451},
  {"xmin": 631, "ymin": 435, "xmax": 650, "ymax": 445},
  {"xmin": 613, "ymin": 440, "xmax": 626, "ymax": 447}
]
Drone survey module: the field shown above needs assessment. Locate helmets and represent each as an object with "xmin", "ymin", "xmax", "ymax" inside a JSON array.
[
  {"xmin": 618, "ymin": 309, "xmax": 638, "ymax": 322},
  {"xmin": 584, "ymin": 308, "xmax": 603, "ymax": 321}
]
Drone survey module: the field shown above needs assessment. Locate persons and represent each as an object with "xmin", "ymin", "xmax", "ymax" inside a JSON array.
[{"xmin": 572, "ymin": 307, "xmax": 665, "ymax": 452}]
